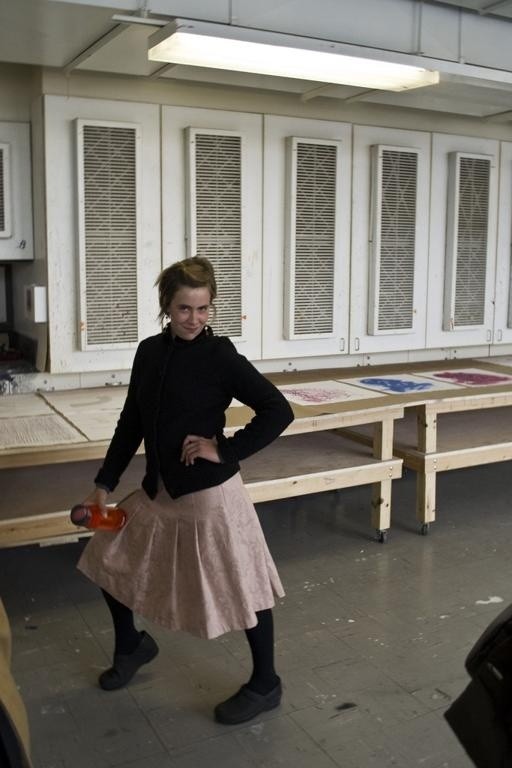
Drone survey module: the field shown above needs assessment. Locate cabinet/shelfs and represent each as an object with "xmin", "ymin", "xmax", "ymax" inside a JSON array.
[
  {"xmin": 426, "ymin": 130, "xmax": 511, "ymax": 363},
  {"xmin": 45, "ymin": 92, "xmax": 263, "ymax": 390},
  {"xmin": 265, "ymin": 110, "xmax": 432, "ymax": 374}
]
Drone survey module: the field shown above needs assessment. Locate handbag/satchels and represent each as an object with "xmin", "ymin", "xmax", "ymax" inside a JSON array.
[{"xmin": 443, "ymin": 602, "xmax": 512, "ymax": 767}]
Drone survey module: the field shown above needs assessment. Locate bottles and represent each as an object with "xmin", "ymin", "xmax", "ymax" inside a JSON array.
[{"xmin": 69, "ymin": 503, "xmax": 126, "ymax": 532}]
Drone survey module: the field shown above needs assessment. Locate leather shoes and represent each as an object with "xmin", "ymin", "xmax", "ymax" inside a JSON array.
[
  {"xmin": 213, "ymin": 678, "xmax": 284, "ymax": 724},
  {"xmin": 98, "ymin": 630, "xmax": 159, "ymax": 690}
]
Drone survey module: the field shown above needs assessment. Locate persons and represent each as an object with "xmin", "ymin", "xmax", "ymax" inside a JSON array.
[{"xmin": 80, "ymin": 256, "xmax": 295, "ymax": 725}]
